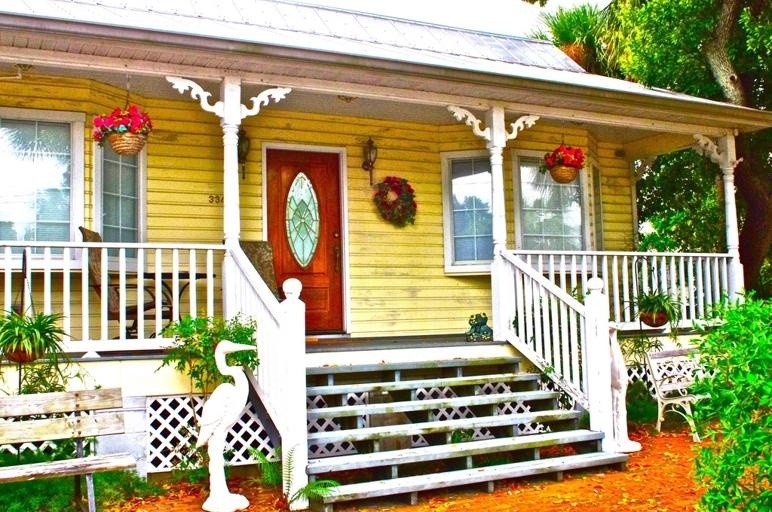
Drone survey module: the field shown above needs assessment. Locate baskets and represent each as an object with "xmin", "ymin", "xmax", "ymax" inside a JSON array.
[
  {"xmin": 4, "ymin": 342, "xmax": 46, "ymax": 364},
  {"xmin": 550, "ymin": 163, "xmax": 578, "ymax": 184},
  {"xmin": 640, "ymin": 309, "xmax": 669, "ymax": 328},
  {"xmin": 104, "ymin": 133, "xmax": 145, "ymax": 156}
]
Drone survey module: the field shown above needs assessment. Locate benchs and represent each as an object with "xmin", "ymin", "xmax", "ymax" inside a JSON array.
[
  {"xmin": 0, "ymin": 388, "xmax": 137, "ymax": 512},
  {"xmin": 644, "ymin": 347, "xmax": 731, "ymax": 443}
]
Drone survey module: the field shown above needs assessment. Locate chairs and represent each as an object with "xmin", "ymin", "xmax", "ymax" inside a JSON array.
[{"xmin": 79, "ymin": 225, "xmax": 173, "ymax": 340}]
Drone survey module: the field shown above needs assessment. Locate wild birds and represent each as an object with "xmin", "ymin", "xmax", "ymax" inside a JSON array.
[{"xmin": 194, "ymin": 339, "xmax": 255, "ymax": 453}]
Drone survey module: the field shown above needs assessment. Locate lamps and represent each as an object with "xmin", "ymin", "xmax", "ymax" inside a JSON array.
[
  {"xmin": 361, "ymin": 137, "xmax": 378, "ymax": 186},
  {"xmin": 236, "ymin": 126, "xmax": 252, "ymax": 180}
]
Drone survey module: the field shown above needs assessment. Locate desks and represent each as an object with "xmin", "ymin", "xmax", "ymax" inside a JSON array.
[{"xmin": 143, "ymin": 268, "xmax": 217, "ymax": 339}]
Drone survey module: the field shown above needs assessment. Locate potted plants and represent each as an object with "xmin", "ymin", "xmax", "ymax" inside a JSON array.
[
  {"xmin": 0, "ymin": 298, "xmax": 101, "ymax": 395},
  {"xmin": 619, "ymin": 287, "xmax": 690, "ymax": 372}
]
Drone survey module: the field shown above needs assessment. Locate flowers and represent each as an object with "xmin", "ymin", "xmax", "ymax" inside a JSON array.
[
  {"xmin": 537, "ymin": 143, "xmax": 585, "ymax": 175},
  {"xmin": 92, "ymin": 102, "xmax": 154, "ymax": 146},
  {"xmin": 372, "ymin": 174, "xmax": 417, "ymax": 229}
]
